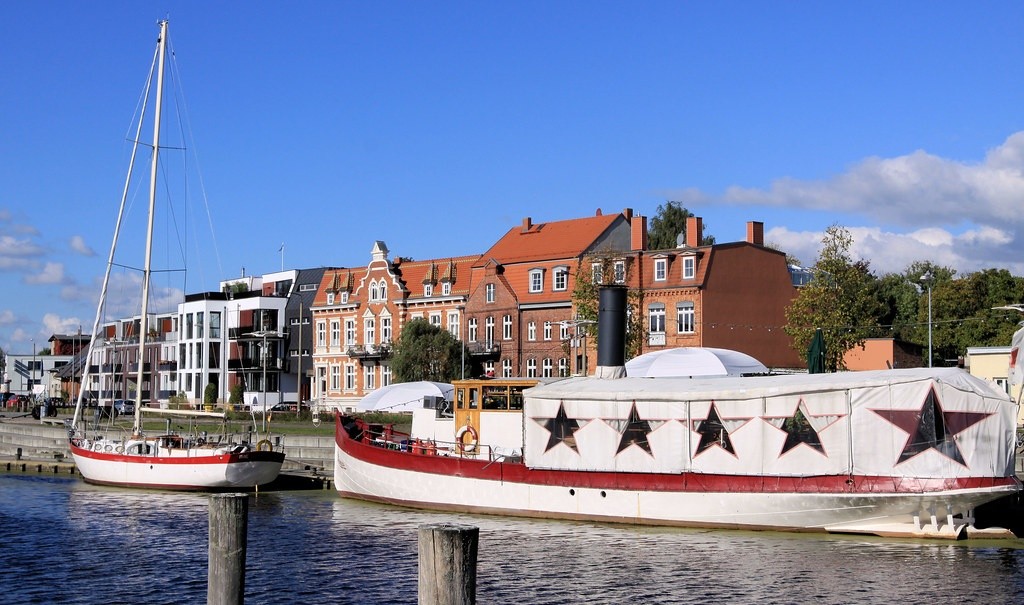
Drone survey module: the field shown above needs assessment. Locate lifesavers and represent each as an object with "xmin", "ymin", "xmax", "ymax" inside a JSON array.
[
  {"xmin": 257, "ymin": 439, "xmax": 273, "ymax": 452},
  {"xmin": 456, "ymin": 426, "xmax": 478, "ymax": 452}
]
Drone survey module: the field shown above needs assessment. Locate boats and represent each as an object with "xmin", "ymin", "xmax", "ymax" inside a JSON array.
[{"xmin": 332, "ymin": 345, "xmax": 1024, "ymax": 538}]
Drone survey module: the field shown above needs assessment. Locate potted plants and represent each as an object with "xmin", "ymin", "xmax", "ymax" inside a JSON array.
[
  {"xmin": 229, "ymin": 384, "xmax": 245, "ymax": 412},
  {"xmin": 204, "ymin": 383, "xmax": 216, "ymax": 411}
]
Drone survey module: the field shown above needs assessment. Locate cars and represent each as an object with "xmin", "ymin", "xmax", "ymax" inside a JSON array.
[
  {"xmin": 114, "ymin": 399, "xmax": 136, "ymax": 415},
  {"xmin": 5, "ymin": 395, "xmax": 30, "ymax": 409},
  {"xmin": 267, "ymin": 400, "xmax": 309, "ymax": 413}
]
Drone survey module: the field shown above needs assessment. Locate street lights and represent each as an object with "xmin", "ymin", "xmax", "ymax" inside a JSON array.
[
  {"xmin": 918, "ymin": 275, "xmax": 933, "ymax": 369},
  {"xmin": 30, "ymin": 337, "xmax": 35, "ymax": 411}
]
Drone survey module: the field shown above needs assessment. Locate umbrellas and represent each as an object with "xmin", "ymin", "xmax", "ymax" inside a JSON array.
[{"xmin": 805, "ymin": 328, "xmax": 827, "ymax": 374}]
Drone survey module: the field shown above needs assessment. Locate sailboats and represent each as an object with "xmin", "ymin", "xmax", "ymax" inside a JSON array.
[{"xmin": 65, "ymin": 17, "xmax": 290, "ymax": 491}]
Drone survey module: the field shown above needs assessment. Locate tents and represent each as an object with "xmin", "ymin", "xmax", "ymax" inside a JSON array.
[
  {"xmin": 625, "ymin": 345, "xmax": 770, "ymax": 376},
  {"xmin": 355, "ymin": 380, "xmax": 480, "ymax": 414}
]
